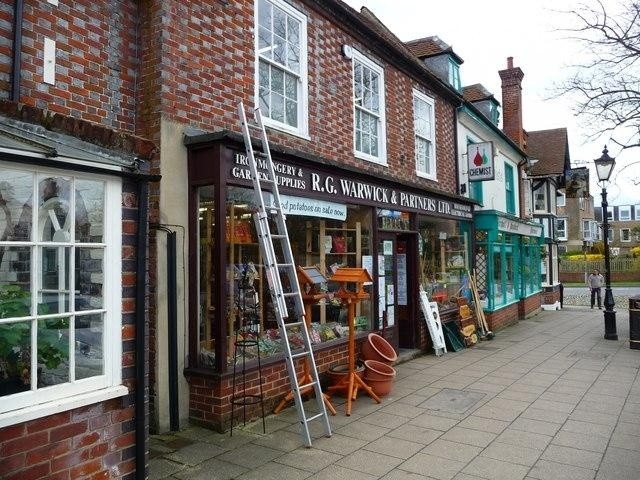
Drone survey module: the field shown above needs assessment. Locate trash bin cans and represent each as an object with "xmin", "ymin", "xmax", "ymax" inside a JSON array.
[{"xmin": 629, "ymin": 296, "xmax": 640, "ymax": 350}]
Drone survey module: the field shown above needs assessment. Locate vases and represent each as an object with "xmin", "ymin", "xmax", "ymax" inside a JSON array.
[{"xmin": 327, "ymin": 333, "xmax": 398, "ymax": 398}]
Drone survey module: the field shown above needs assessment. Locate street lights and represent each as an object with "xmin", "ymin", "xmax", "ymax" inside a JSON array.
[{"xmin": 593, "ymin": 143, "xmax": 618, "ymax": 341}]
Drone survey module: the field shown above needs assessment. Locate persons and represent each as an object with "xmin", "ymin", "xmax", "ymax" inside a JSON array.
[{"xmin": 588, "ymin": 269, "xmax": 604, "ymax": 309}]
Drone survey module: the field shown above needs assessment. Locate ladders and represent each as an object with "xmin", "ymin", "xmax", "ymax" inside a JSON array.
[{"xmin": 236, "ymin": 102, "xmax": 333, "ymax": 449}]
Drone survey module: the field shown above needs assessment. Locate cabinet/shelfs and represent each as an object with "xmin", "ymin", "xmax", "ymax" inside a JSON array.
[
  {"xmin": 428, "ymin": 232, "xmax": 469, "ymax": 298},
  {"xmin": 305, "ymin": 219, "xmax": 361, "ymax": 323},
  {"xmin": 207, "ymin": 203, "xmax": 264, "ymax": 358}
]
